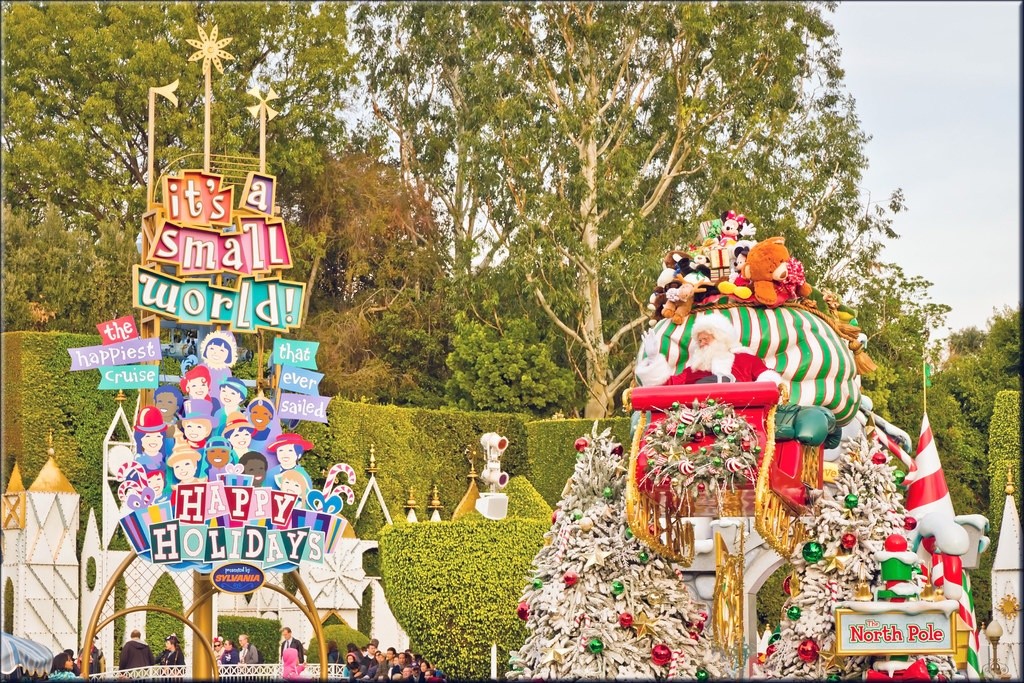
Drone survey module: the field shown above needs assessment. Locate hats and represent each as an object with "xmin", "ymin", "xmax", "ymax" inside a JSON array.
[
  {"xmin": 345, "ymin": 653, "xmax": 357, "ymax": 662},
  {"xmin": 378, "ymin": 652, "xmax": 387, "ymax": 658},
  {"xmin": 366, "ymin": 639, "xmax": 379, "ymax": 649}
]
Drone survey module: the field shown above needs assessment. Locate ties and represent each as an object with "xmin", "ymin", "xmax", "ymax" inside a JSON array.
[{"xmin": 287, "ymin": 641, "xmax": 289, "ymax": 649}]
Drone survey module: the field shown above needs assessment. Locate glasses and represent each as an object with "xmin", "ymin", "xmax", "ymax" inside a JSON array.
[{"xmin": 215, "ymin": 645, "xmax": 220, "ymax": 647}]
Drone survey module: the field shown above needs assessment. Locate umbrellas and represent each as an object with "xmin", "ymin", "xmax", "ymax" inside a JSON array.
[{"xmin": 0, "ymin": 629, "xmax": 54, "ymax": 678}]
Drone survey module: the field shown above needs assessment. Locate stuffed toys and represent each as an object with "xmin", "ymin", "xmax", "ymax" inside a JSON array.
[{"xmin": 649, "ymin": 210, "xmax": 813, "ymax": 324}]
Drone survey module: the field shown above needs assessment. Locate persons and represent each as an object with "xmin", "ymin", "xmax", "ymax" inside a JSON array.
[
  {"xmin": 281, "ymin": 627, "xmax": 304, "ymax": 664},
  {"xmin": 239, "ymin": 635, "xmax": 258, "ymax": 683},
  {"xmin": 52, "ymin": 634, "xmax": 106, "ymax": 683},
  {"xmin": 119, "ymin": 629, "xmax": 155, "ymax": 683},
  {"xmin": 327, "ymin": 641, "xmax": 344, "ymax": 664},
  {"xmin": 214, "ymin": 637, "xmax": 225, "ymax": 660},
  {"xmin": 154, "ymin": 636, "xmax": 186, "ymax": 683},
  {"xmin": 283, "ymin": 648, "xmax": 309, "ymax": 680},
  {"xmin": 343, "ymin": 639, "xmax": 449, "ymax": 681},
  {"xmin": 216, "ymin": 640, "xmax": 240, "ymax": 683},
  {"xmin": 634, "ymin": 312, "xmax": 791, "ymax": 405}
]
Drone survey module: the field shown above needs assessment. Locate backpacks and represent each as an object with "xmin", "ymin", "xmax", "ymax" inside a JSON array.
[{"xmin": 247, "ymin": 643, "xmax": 264, "ymax": 664}]
